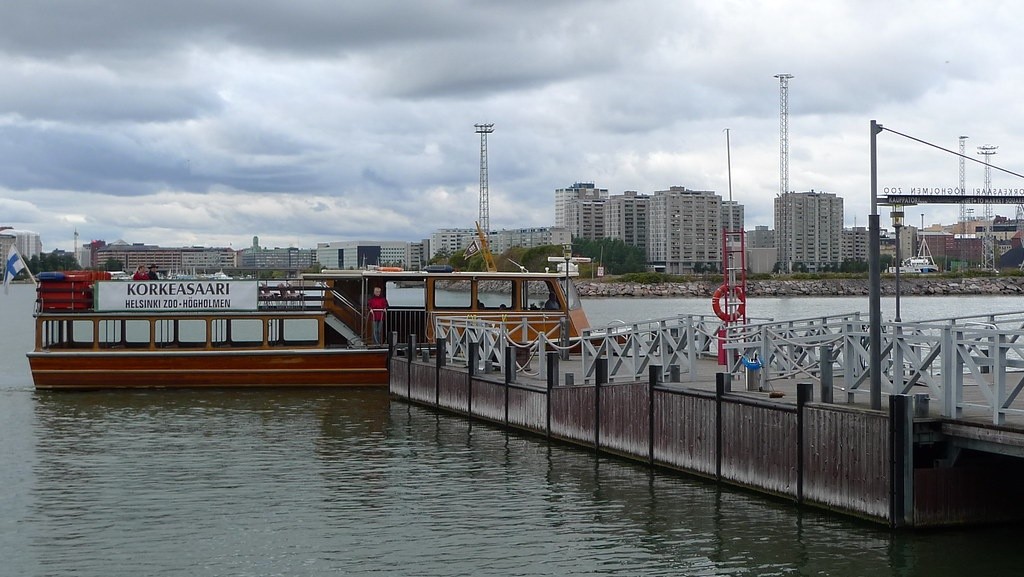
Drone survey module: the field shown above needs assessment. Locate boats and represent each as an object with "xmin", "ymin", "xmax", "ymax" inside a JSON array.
[
  {"xmin": 881, "ymin": 236, "xmax": 940, "ymax": 274},
  {"xmin": 25, "ymin": 263, "xmax": 641, "ymax": 394}
]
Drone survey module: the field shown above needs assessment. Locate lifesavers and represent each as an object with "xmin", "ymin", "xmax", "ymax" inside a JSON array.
[{"xmin": 711, "ymin": 284, "xmax": 746, "ymax": 321}]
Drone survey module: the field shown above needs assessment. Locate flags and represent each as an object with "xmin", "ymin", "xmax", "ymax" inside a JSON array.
[{"xmin": 3, "ymin": 243, "xmax": 26, "ymax": 297}]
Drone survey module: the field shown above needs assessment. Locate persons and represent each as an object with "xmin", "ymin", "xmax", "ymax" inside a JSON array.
[
  {"xmin": 369, "ymin": 287, "xmax": 388, "ymax": 344},
  {"xmin": 477, "ymin": 301, "xmax": 538, "ymax": 310},
  {"xmin": 147, "ymin": 263, "xmax": 160, "ymax": 280},
  {"xmin": 133, "ymin": 266, "xmax": 149, "ymax": 281},
  {"xmin": 545, "ymin": 292, "xmax": 560, "ymax": 310}
]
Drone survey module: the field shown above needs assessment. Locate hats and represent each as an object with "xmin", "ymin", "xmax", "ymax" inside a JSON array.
[{"xmin": 147, "ymin": 264, "xmax": 159, "ymax": 269}]
